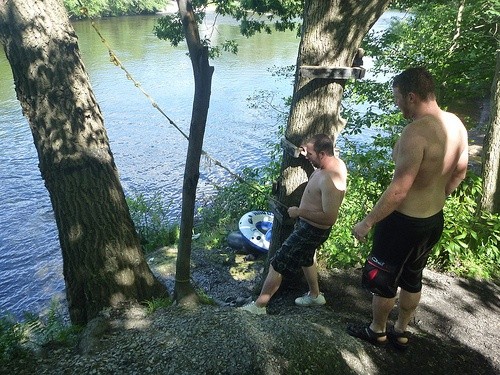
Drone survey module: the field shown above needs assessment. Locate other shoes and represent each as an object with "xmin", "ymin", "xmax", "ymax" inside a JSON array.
[
  {"xmin": 237, "ymin": 300, "xmax": 267, "ymax": 315},
  {"xmin": 294, "ymin": 291, "xmax": 327, "ymax": 306}
]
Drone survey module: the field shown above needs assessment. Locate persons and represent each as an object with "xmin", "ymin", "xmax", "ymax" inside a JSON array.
[
  {"xmin": 347, "ymin": 65, "xmax": 468, "ymax": 352},
  {"xmin": 238, "ymin": 134, "xmax": 348, "ymax": 316}
]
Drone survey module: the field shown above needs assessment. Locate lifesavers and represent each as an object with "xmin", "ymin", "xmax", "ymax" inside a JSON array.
[{"xmin": 238, "ymin": 210, "xmax": 274, "ymax": 254}]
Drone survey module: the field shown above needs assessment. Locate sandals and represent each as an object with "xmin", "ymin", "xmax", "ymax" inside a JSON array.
[
  {"xmin": 345, "ymin": 320, "xmax": 389, "ymax": 347},
  {"xmin": 386, "ymin": 325, "xmax": 411, "ymax": 346}
]
